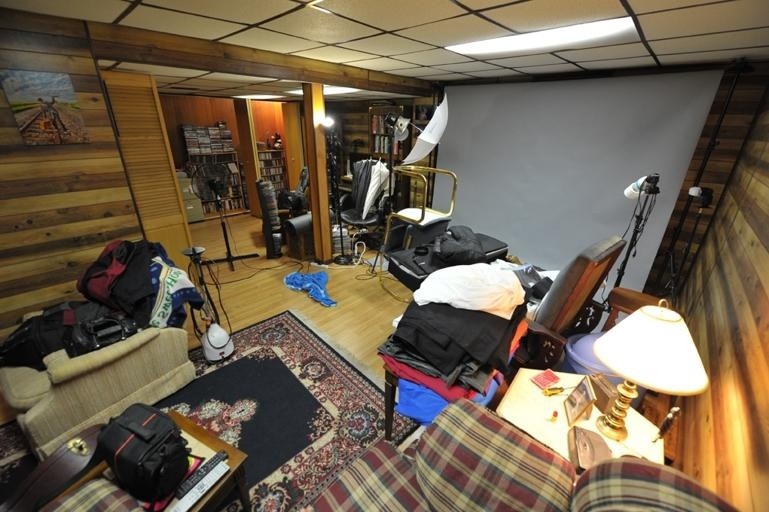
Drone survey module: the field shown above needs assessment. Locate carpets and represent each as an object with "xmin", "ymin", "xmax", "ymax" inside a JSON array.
[{"xmin": 0, "ymin": 308, "xmax": 421, "ymax": 512}]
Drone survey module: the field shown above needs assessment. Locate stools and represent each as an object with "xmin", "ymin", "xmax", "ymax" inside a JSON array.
[
  {"xmin": 601, "ymin": 287, "xmax": 672, "ymax": 333},
  {"xmin": 382, "ymin": 367, "xmax": 519, "ymax": 442}
]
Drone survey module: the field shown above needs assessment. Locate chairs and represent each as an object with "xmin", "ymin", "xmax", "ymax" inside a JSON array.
[
  {"xmin": 0, "ymin": 310, "xmax": 196, "ymax": 463},
  {"xmin": 303, "ymin": 397, "xmax": 738, "ymax": 512},
  {"xmin": 339, "ymin": 160, "xmax": 388, "ymax": 250},
  {"xmin": 510, "ymin": 235, "xmax": 627, "ymax": 370},
  {"xmin": 262, "ymin": 168, "xmax": 309, "ymax": 233}
]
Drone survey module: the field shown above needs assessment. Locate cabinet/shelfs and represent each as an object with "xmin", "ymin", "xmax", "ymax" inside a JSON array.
[
  {"xmin": 189, "ymin": 149, "xmax": 289, "ymax": 221},
  {"xmin": 368, "ymin": 105, "xmax": 413, "ymax": 212}
]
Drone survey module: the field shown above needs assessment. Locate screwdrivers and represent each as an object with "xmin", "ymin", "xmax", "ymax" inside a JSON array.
[{"xmin": 544, "ymin": 387, "xmax": 577, "ymax": 396}]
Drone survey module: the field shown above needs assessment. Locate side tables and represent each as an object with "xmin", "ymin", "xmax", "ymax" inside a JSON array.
[{"xmin": 495, "ymin": 367, "xmax": 665, "ymax": 476}]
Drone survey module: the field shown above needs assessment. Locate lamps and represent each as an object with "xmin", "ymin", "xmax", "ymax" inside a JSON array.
[
  {"xmin": 384, "ymin": 111, "xmax": 411, "ymax": 213},
  {"xmin": 593, "ymin": 304, "xmax": 709, "ymax": 441},
  {"xmin": 607, "ymin": 173, "xmax": 660, "ymax": 313},
  {"xmin": 667, "ymin": 186, "xmax": 714, "ymax": 302},
  {"xmin": 321, "ymin": 116, "xmax": 352, "ymax": 265}
]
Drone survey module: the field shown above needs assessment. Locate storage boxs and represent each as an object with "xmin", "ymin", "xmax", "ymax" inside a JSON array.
[{"xmin": 178, "ymin": 178, "xmax": 204, "ymax": 223}]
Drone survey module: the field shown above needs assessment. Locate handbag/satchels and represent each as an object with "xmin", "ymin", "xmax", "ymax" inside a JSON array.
[
  {"xmin": 77, "ymin": 239, "xmax": 136, "ymax": 311},
  {"xmin": 99, "ymin": 402, "xmax": 192, "ymax": 502},
  {"xmin": 1, "ymin": 299, "xmax": 137, "ymax": 373}
]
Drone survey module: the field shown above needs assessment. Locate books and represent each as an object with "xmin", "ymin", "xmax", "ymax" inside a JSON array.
[{"xmin": 182, "ymin": 124, "xmax": 288, "ymax": 214}]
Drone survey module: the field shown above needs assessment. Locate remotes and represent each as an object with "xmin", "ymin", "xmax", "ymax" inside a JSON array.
[
  {"xmin": 175, "ymin": 449, "xmax": 228, "ymax": 500},
  {"xmin": 169, "ymin": 460, "xmax": 230, "ymax": 512}
]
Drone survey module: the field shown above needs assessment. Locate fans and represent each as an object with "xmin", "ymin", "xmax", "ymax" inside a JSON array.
[{"xmin": 191, "ymin": 161, "xmax": 259, "ymax": 271}]
